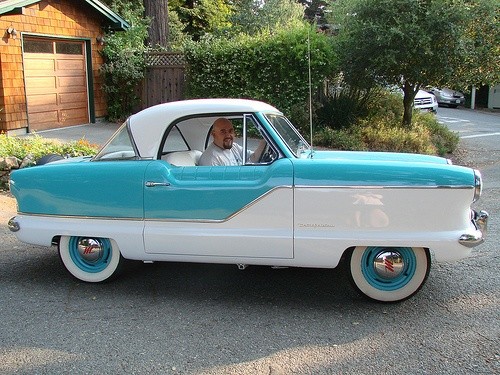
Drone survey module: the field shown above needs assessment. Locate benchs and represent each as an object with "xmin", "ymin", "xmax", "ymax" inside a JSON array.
[{"xmin": 163, "ymin": 149, "xmax": 203, "ymax": 166}]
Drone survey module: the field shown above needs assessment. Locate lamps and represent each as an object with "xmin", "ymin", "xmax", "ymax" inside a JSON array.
[
  {"xmin": 7, "ymin": 28, "xmax": 18, "ymax": 36},
  {"xmin": 97, "ymin": 34, "xmax": 104, "ymax": 43}
]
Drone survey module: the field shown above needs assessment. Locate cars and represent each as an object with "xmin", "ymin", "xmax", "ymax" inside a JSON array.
[
  {"xmin": 8, "ymin": 97, "xmax": 488, "ymax": 303},
  {"xmin": 396, "ymin": 83, "xmax": 466, "ymax": 113}
]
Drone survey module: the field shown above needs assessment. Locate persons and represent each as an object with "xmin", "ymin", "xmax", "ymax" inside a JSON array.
[{"xmin": 199, "ymin": 118, "xmax": 269, "ymax": 166}]
